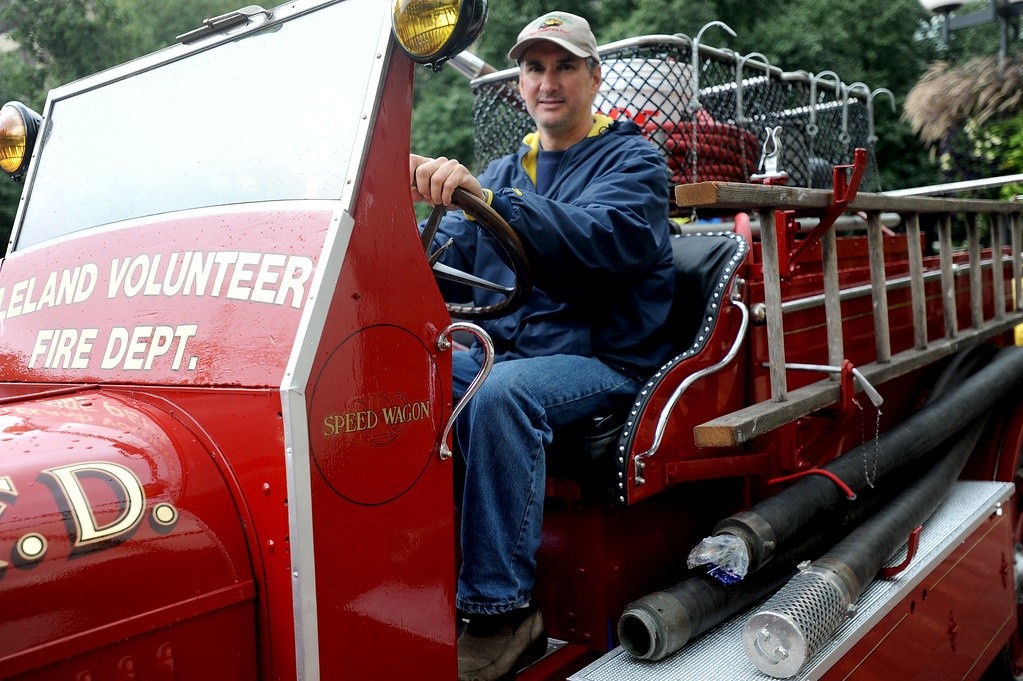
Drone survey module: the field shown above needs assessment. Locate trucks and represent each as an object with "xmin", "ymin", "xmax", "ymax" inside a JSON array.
[{"xmin": 0, "ymin": 1, "xmax": 1022, "ymax": 680}]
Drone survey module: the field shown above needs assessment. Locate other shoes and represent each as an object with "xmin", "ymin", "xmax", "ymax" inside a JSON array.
[{"xmin": 456, "ymin": 608, "xmax": 547, "ymax": 681}]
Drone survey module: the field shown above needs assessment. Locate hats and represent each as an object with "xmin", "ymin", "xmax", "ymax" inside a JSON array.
[{"xmin": 508, "ymin": 12, "xmax": 600, "ymax": 65}]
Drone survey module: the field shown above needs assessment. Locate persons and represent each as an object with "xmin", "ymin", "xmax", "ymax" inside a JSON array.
[{"xmin": 410, "ymin": 11, "xmax": 674, "ymax": 681}]
